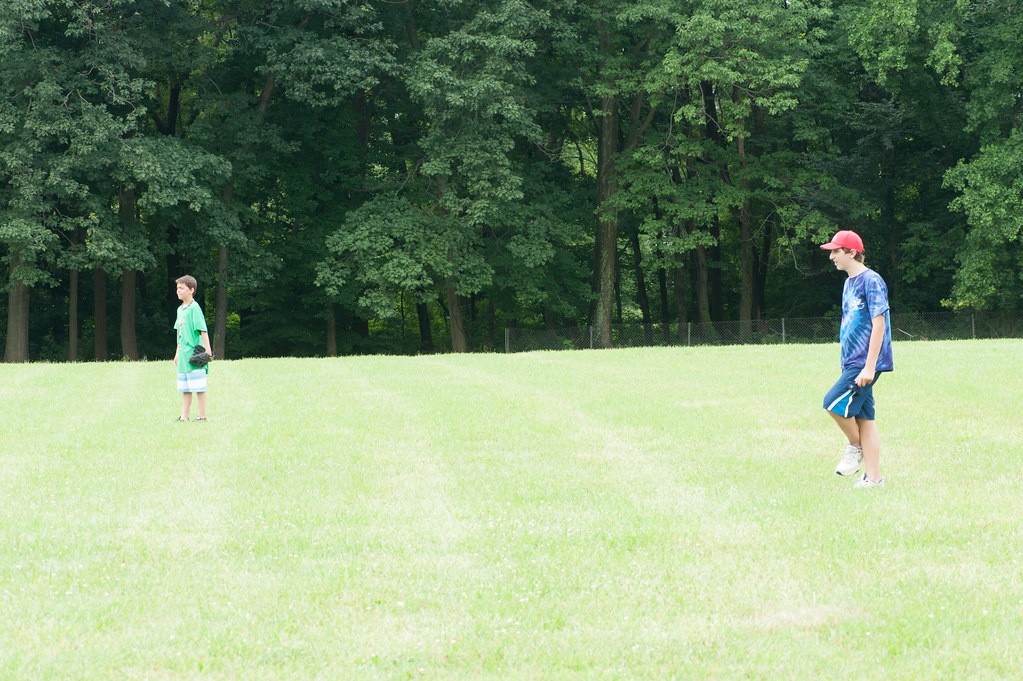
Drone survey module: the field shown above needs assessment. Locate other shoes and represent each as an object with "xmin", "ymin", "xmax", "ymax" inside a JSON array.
[
  {"xmin": 192, "ymin": 416, "xmax": 207, "ymax": 423},
  {"xmin": 177, "ymin": 416, "xmax": 190, "ymax": 422}
]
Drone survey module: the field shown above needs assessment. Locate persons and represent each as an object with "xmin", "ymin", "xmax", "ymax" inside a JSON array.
[
  {"xmin": 820, "ymin": 228, "xmax": 893, "ymax": 489},
  {"xmin": 174, "ymin": 274, "xmax": 211, "ymax": 422}
]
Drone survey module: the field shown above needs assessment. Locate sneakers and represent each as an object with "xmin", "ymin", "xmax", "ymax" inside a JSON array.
[
  {"xmin": 836, "ymin": 442, "xmax": 863, "ymax": 476},
  {"xmin": 855, "ymin": 475, "xmax": 885, "ymax": 491}
]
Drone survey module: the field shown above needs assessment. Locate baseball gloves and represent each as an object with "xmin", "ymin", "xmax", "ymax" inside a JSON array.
[{"xmin": 190, "ymin": 345, "xmax": 211, "ymax": 367}]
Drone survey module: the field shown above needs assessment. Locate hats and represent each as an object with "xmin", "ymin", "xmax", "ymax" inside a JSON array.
[{"xmin": 820, "ymin": 230, "xmax": 864, "ymax": 254}]
